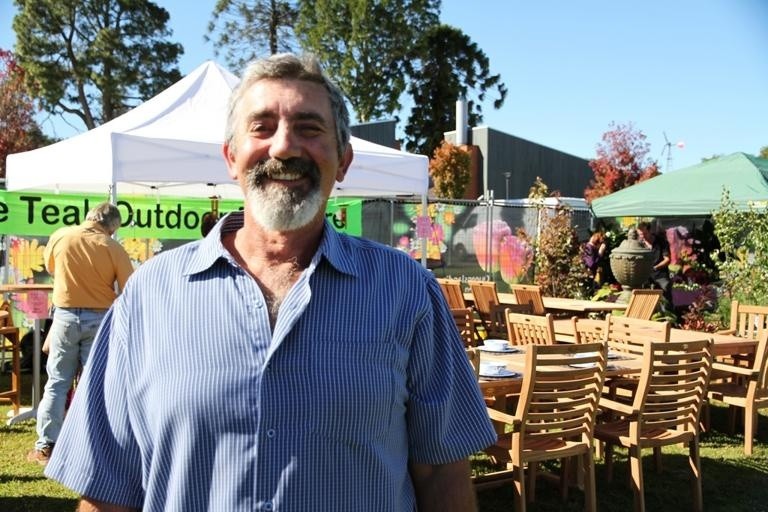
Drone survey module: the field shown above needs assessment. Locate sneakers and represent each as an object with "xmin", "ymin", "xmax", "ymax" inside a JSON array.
[{"xmin": 37, "ymin": 447, "xmax": 50, "ymax": 465}]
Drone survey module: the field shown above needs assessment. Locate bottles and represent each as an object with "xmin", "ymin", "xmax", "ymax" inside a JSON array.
[{"xmin": 573, "ymin": 292, "xmax": 584, "ymax": 306}]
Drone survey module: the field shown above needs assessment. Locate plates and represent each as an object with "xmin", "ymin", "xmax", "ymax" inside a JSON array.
[
  {"xmin": 478, "ymin": 346, "xmax": 517, "ymax": 353},
  {"xmin": 478, "ymin": 370, "xmax": 515, "ymax": 378},
  {"xmin": 568, "ymin": 350, "xmax": 622, "ymax": 371}
]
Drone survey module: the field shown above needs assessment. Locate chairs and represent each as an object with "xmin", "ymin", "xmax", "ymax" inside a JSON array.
[
  {"xmin": 435, "ymin": 278, "xmax": 768, "ymax": 510},
  {"xmin": 0, "ymin": 307, "xmax": 20, "ymax": 417}
]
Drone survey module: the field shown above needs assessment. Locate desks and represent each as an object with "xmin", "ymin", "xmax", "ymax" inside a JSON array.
[{"xmin": 3, "ymin": 283, "xmax": 52, "ymax": 425}]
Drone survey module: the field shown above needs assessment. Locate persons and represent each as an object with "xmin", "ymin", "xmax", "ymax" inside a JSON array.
[
  {"xmin": 27, "ymin": 202, "xmax": 134, "ymax": 465},
  {"xmin": 582, "ymin": 219, "xmax": 726, "ymax": 316},
  {"xmin": 44, "ymin": 51, "xmax": 497, "ymax": 512}
]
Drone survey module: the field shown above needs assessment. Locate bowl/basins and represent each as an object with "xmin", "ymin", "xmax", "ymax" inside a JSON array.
[
  {"xmin": 480, "ymin": 360, "xmax": 507, "ymax": 375},
  {"xmin": 483, "ymin": 340, "xmax": 507, "ymax": 350}
]
